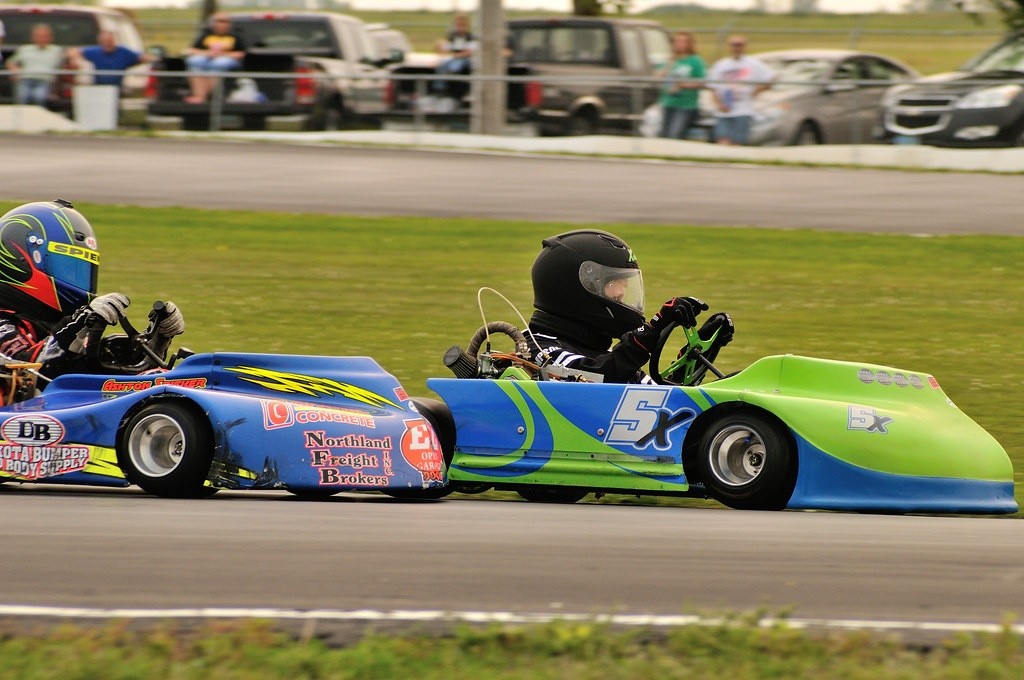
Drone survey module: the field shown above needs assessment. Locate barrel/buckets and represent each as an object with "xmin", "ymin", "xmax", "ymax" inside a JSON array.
[{"xmin": 71, "ymin": 85, "xmax": 117, "ymax": 129}]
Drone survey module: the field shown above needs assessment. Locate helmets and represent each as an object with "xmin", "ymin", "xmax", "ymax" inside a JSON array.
[
  {"xmin": 1, "ymin": 198, "xmax": 102, "ymax": 323},
  {"xmin": 528, "ymin": 228, "xmax": 646, "ymax": 353}
]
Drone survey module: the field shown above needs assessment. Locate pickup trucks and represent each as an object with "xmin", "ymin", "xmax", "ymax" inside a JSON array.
[{"xmin": 145, "ymin": 11, "xmax": 405, "ymax": 131}]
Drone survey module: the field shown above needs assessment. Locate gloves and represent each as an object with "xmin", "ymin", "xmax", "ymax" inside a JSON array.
[
  {"xmin": 677, "ymin": 311, "xmax": 735, "ymax": 370},
  {"xmin": 145, "ymin": 299, "xmax": 187, "ymax": 338},
  {"xmin": 50, "ymin": 292, "xmax": 131, "ymax": 355},
  {"xmin": 631, "ymin": 294, "xmax": 710, "ymax": 354}
]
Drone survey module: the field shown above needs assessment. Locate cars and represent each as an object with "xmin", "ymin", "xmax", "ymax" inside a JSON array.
[
  {"xmin": 880, "ymin": 33, "xmax": 1024, "ymax": 147},
  {"xmin": 0, "ymin": 5, "xmax": 165, "ymax": 112},
  {"xmin": 640, "ymin": 49, "xmax": 922, "ymax": 147}
]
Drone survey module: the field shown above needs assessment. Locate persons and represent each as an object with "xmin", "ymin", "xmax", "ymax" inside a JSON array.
[
  {"xmin": 515, "ymin": 229, "xmax": 735, "ymax": 385},
  {"xmin": 433, "ymin": 14, "xmax": 479, "ymax": 96},
  {"xmin": 182, "ymin": 12, "xmax": 245, "ymax": 104},
  {"xmin": 0, "ymin": 200, "xmax": 185, "ymax": 407},
  {"xmin": 66, "ymin": 31, "xmax": 162, "ymax": 97},
  {"xmin": 708, "ymin": 34, "xmax": 777, "ymax": 146},
  {"xmin": 5, "ymin": 21, "xmax": 64, "ymax": 104},
  {"xmin": 652, "ymin": 29, "xmax": 705, "ymax": 139}
]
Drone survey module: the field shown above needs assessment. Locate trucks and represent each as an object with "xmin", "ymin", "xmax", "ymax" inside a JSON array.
[{"xmin": 383, "ymin": 19, "xmax": 679, "ymax": 137}]
